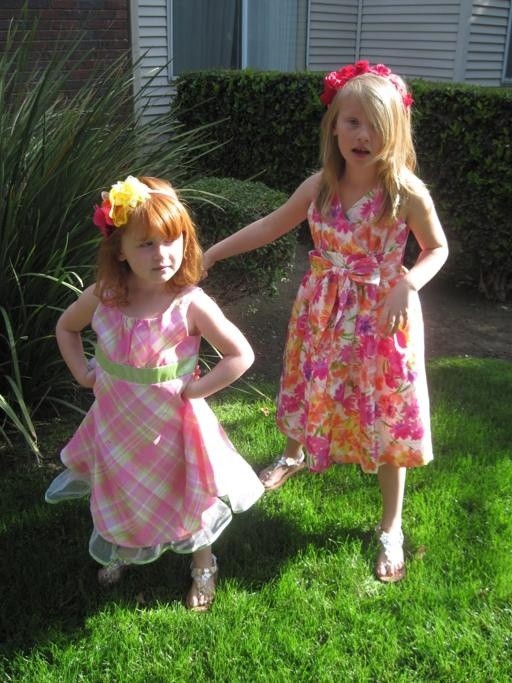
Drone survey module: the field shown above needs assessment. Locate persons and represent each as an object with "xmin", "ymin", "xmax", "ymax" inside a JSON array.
[
  {"xmin": 201, "ymin": 61, "xmax": 450, "ymax": 583},
  {"xmin": 45, "ymin": 177, "xmax": 265, "ymax": 612}
]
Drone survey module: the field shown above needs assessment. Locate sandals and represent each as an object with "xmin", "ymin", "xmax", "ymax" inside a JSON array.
[
  {"xmin": 259, "ymin": 450, "xmax": 306, "ymax": 490},
  {"xmin": 186, "ymin": 553, "xmax": 219, "ymax": 611},
  {"xmin": 97, "ymin": 562, "xmax": 125, "ymax": 586},
  {"xmin": 375, "ymin": 523, "xmax": 405, "ymax": 582}
]
